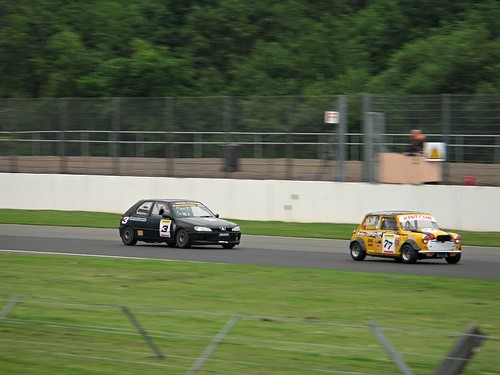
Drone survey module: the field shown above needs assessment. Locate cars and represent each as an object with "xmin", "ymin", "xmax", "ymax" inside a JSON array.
[
  {"xmin": 349, "ymin": 211, "xmax": 462, "ymax": 265},
  {"xmin": 118, "ymin": 199, "xmax": 242, "ymax": 248}
]
